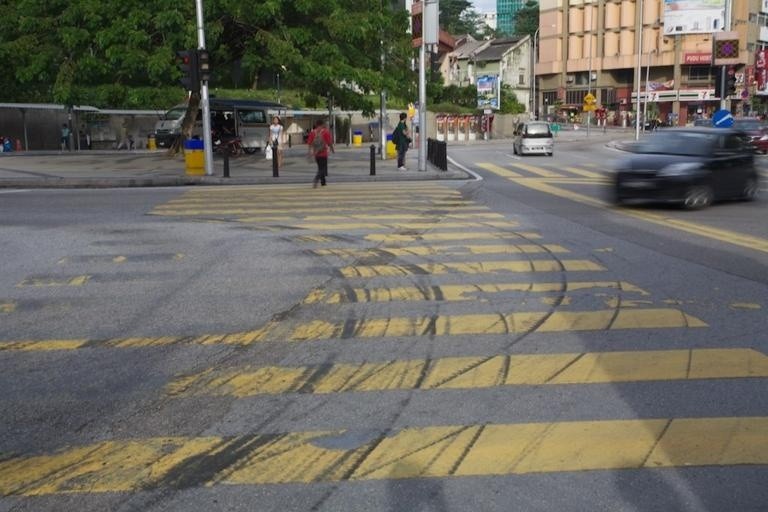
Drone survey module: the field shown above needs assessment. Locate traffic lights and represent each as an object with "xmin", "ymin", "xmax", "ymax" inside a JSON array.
[{"xmin": 174, "ymin": 51, "xmax": 201, "ymax": 94}]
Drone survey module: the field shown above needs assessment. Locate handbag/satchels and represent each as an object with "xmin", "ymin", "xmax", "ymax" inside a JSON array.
[{"xmin": 266, "ymin": 145, "xmax": 272, "ymax": 160}]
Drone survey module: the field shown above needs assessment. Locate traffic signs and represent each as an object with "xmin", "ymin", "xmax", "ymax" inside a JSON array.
[{"xmin": 712, "ymin": 110, "xmax": 734, "ymax": 128}]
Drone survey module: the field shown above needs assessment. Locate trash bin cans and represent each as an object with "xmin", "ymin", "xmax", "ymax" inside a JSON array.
[
  {"xmin": 184, "ymin": 140, "xmax": 206, "ymax": 175},
  {"xmin": 387, "ymin": 134, "xmax": 398, "ymax": 159},
  {"xmin": 148, "ymin": 133, "xmax": 157, "ymax": 150},
  {"xmin": 352, "ymin": 130, "xmax": 362, "ymax": 146}
]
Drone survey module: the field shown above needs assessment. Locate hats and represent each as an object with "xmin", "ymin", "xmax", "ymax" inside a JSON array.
[{"xmin": 272, "ymin": 116, "xmax": 281, "ymax": 122}]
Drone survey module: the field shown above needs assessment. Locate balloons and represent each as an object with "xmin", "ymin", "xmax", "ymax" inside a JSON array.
[{"xmin": 407, "ymin": 102, "xmax": 415, "ymax": 119}]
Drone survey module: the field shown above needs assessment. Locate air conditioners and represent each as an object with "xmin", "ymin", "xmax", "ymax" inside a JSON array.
[
  {"xmin": 566, "ymin": 76, "xmax": 574, "ymax": 82},
  {"xmin": 592, "ymin": 73, "xmax": 596, "ymax": 79}
]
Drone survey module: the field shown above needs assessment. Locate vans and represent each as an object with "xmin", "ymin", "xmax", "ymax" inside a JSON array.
[
  {"xmin": 513, "ymin": 120, "xmax": 554, "ymax": 156},
  {"xmin": 154, "ymin": 97, "xmax": 286, "ymax": 154}
]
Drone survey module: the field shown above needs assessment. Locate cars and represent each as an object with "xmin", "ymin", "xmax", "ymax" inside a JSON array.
[
  {"xmin": 748, "ymin": 128, "xmax": 767, "ymax": 154},
  {"xmin": 617, "ymin": 130, "xmax": 756, "ymax": 211}
]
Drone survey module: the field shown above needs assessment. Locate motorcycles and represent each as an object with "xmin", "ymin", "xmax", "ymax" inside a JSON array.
[{"xmin": 211, "ymin": 125, "xmax": 244, "ymax": 158}]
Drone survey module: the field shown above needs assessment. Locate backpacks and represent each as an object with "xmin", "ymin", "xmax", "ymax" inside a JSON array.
[
  {"xmin": 392, "ymin": 128, "xmax": 399, "ymax": 144},
  {"xmin": 313, "ymin": 129, "xmax": 324, "ymax": 150}
]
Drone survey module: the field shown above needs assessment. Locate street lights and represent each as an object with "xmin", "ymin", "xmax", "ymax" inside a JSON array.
[
  {"xmin": 642, "ymin": 50, "xmax": 667, "ymax": 132},
  {"xmin": 533, "ymin": 24, "xmax": 555, "ymax": 124}
]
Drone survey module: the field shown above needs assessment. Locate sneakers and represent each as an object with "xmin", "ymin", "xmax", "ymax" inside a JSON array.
[{"xmin": 398, "ymin": 166, "xmax": 408, "ymax": 171}]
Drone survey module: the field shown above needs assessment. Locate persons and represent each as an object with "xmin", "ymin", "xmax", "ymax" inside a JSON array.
[
  {"xmin": 60, "ymin": 122, "xmax": 74, "ymax": 153},
  {"xmin": 306, "ymin": 118, "xmax": 336, "ymax": 188},
  {"xmin": 116, "ymin": 123, "xmax": 131, "ymax": 152},
  {"xmin": 395, "ymin": 112, "xmax": 411, "ymax": 171},
  {"xmin": 268, "ymin": 116, "xmax": 284, "ymax": 168},
  {"xmin": 211, "ymin": 111, "xmax": 235, "ymax": 145},
  {"xmin": 79, "ymin": 123, "xmax": 88, "ymax": 145}
]
